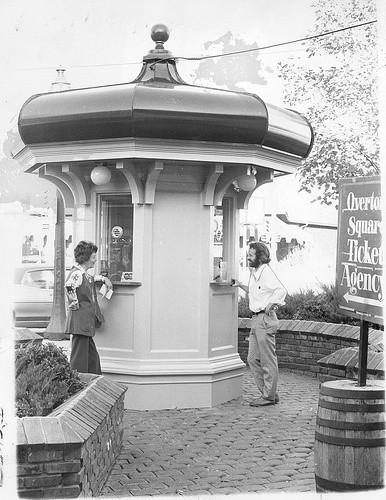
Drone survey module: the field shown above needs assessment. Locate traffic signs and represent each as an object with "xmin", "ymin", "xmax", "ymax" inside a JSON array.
[{"xmin": 334, "ymin": 175, "xmax": 385, "ymax": 326}]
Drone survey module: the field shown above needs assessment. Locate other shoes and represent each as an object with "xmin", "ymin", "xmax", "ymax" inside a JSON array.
[{"xmin": 249, "ymin": 397, "xmax": 279, "ymax": 407}]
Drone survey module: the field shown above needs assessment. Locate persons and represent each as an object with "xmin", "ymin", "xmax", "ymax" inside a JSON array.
[
  {"xmin": 230, "ymin": 241, "xmax": 287, "ymax": 406},
  {"xmin": 64, "ymin": 240, "xmax": 113, "ymax": 374}
]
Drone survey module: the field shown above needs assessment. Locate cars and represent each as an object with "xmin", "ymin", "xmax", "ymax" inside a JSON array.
[{"xmin": 12, "ymin": 265, "xmax": 74, "ymax": 328}]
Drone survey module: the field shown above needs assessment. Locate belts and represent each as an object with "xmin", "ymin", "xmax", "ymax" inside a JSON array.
[{"xmin": 252, "ymin": 310, "xmax": 266, "ymax": 316}]
